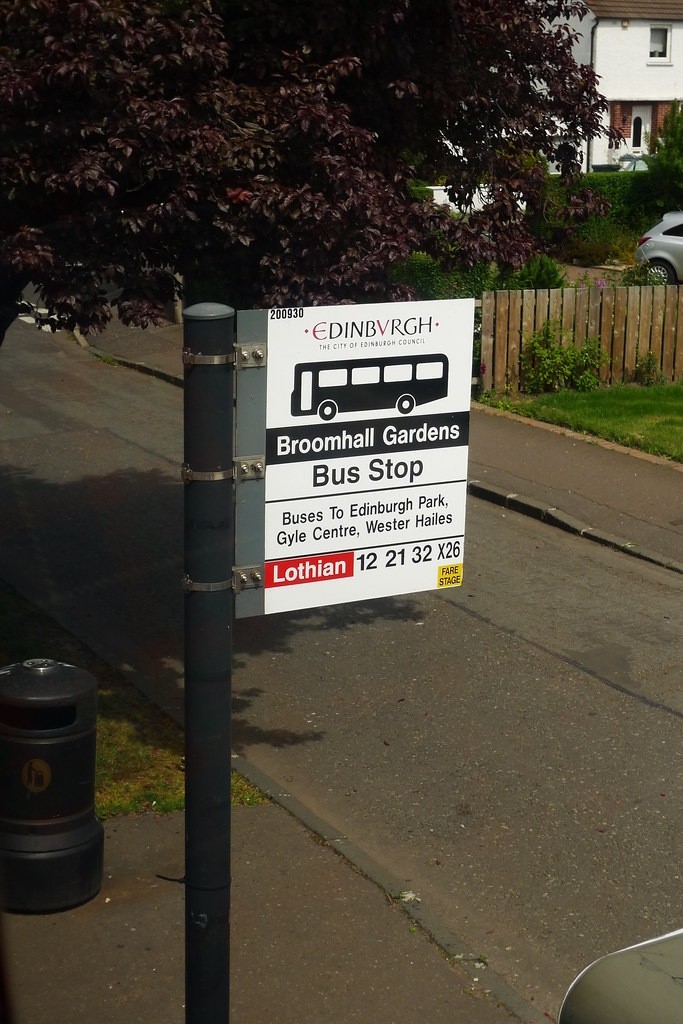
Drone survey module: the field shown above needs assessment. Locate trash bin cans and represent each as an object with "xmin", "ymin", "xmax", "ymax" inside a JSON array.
[{"xmin": 0, "ymin": 657, "xmax": 106, "ymax": 916}]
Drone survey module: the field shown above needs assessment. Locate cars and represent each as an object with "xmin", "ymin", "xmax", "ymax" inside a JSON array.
[{"xmin": 638, "ymin": 210, "xmax": 683, "ymax": 285}]
